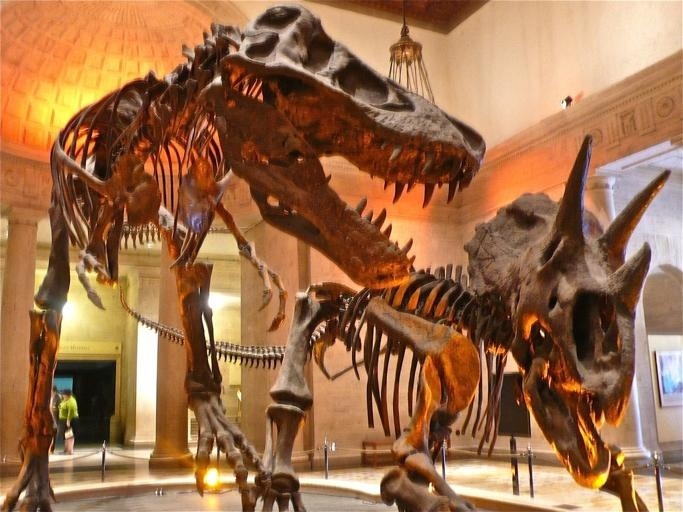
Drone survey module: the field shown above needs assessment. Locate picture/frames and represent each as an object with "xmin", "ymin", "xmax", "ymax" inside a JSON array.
[{"xmin": 653, "ymin": 349, "xmax": 682, "ymax": 409}]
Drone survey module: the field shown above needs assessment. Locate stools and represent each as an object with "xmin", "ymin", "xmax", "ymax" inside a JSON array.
[{"xmin": 360, "ymin": 435, "xmax": 400, "ymax": 468}]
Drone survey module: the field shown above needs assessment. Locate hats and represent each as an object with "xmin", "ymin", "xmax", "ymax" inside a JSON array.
[{"xmin": 59, "ymin": 388, "xmax": 73, "ymax": 395}]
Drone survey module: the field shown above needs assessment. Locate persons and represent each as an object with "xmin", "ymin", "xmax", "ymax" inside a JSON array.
[
  {"xmin": 48, "ymin": 387, "xmax": 62, "ymax": 455},
  {"xmin": 61, "ymin": 389, "xmax": 80, "ymax": 455}
]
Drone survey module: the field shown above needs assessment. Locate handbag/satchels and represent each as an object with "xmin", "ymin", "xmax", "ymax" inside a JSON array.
[{"xmin": 63, "ymin": 427, "xmax": 74, "ymax": 441}]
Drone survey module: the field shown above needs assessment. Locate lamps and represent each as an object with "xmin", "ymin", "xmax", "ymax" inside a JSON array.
[{"xmin": 385, "ymin": 0, "xmax": 435, "ymax": 104}]
